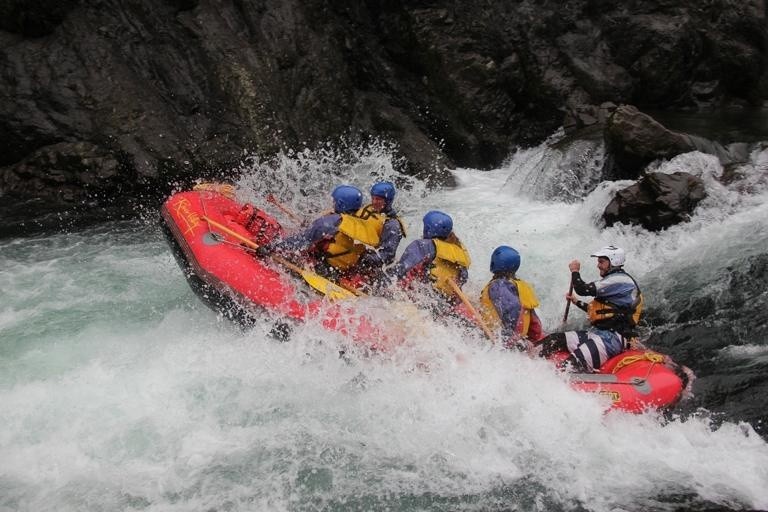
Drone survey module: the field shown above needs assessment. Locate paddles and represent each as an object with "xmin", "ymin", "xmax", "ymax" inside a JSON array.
[{"xmin": 201, "ymin": 213, "xmax": 357, "ymax": 300}]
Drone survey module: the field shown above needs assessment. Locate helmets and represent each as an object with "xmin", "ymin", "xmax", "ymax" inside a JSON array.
[
  {"xmin": 489, "ymin": 246, "xmax": 522, "ymax": 274},
  {"xmin": 423, "ymin": 209, "xmax": 452, "ymax": 240},
  {"xmin": 372, "ymin": 180, "xmax": 397, "ymax": 202},
  {"xmin": 591, "ymin": 245, "xmax": 626, "ymax": 269},
  {"xmin": 332, "ymin": 186, "xmax": 364, "ymax": 214}
]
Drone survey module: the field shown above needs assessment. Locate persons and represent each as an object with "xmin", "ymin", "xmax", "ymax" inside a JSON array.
[
  {"xmin": 256, "ymin": 186, "xmax": 379, "ymax": 285},
  {"xmin": 371, "ymin": 211, "xmax": 470, "ymax": 319},
  {"xmin": 529, "ymin": 244, "xmax": 644, "ymax": 374},
  {"xmin": 478, "ymin": 246, "xmax": 541, "ymax": 352},
  {"xmin": 324, "ymin": 182, "xmax": 407, "ymax": 270}
]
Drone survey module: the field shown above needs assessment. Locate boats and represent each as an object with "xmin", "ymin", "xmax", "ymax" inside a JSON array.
[{"xmin": 159, "ymin": 187, "xmax": 689, "ymax": 423}]
